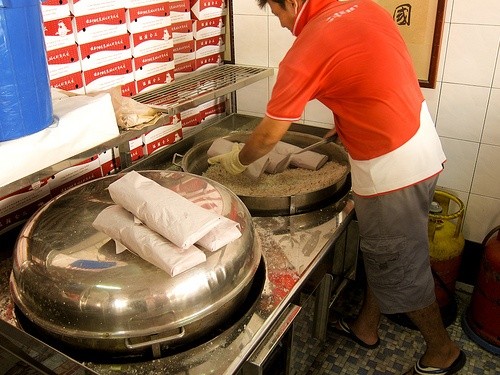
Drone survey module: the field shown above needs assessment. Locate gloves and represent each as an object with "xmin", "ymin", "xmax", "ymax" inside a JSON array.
[{"xmin": 207, "ymin": 142, "xmax": 248, "ymax": 175}]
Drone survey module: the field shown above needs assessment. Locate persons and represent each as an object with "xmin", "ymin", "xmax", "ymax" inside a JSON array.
[{"xmin": 208, "ymin": 0, "xmax": 468, "ymax": 375}]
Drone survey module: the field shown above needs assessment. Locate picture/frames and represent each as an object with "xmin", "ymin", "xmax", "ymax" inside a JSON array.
[{"xmin": 373, "ymin": 0, "xmax": 448, "ymax": 90}]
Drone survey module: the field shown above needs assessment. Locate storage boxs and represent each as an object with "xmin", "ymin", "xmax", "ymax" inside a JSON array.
[{"xmin": 1, "ymin": 1, "xmax": 228, "ymax": 223}]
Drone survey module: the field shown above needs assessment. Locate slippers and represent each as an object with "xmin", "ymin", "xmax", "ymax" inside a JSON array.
[
  {"xmin": 403, "ymin": 350, "xmax": 466, "ymax": 375},
  {"xmin": 327, "ymin": 316, "xmax": 380, "ymax": 349}
]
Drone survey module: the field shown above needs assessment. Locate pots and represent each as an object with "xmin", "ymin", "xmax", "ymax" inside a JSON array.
[
  {"xmin": 7, "ymin": 170, "xmax": 263, "ymax": 360},
  {"xmin": 172, "ymin": 131, "xmax": 351, "ymax": 216}
]
